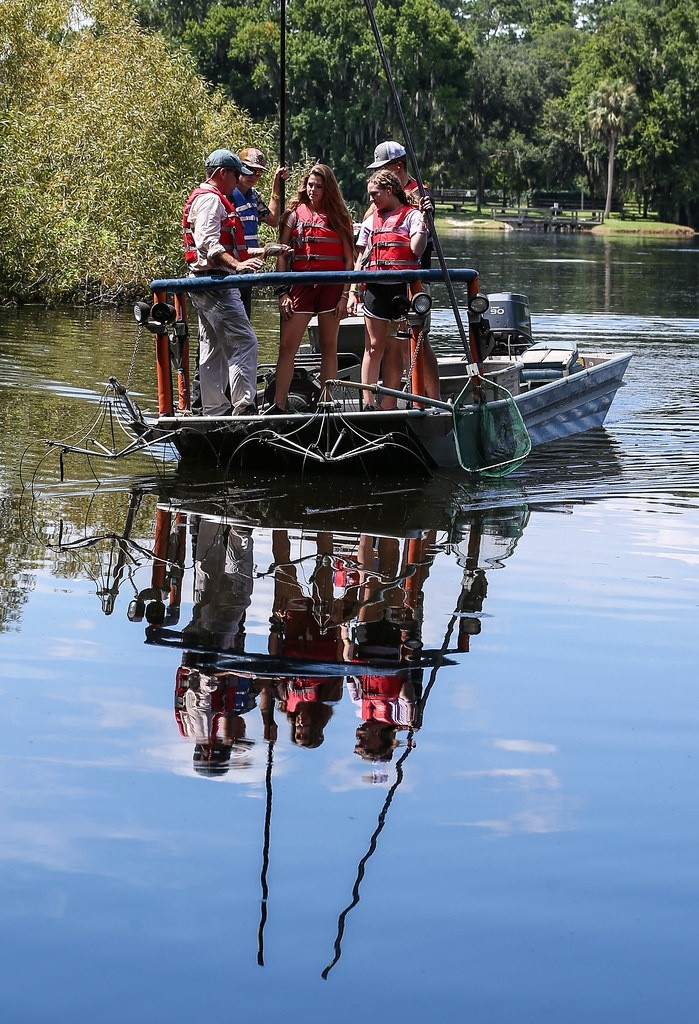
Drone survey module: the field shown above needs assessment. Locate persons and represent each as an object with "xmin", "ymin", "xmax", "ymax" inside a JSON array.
[
  {"xmin": 341, "ymin": 531, "xmax": 439, "ymax": 763},
  {"xmin": 182, "ymin": 149, "xmax": 268, "ymax": 415},
  {"xmin": 190, "ymin": 148, "xmax": 294, "ymax": 416},
  {"xmin": 257, "ymin": 529, "xmax": 346, "ymax": 749},
  {"xmin": 346, "ymin": 169, "xmax": 434, "ymax": 411},
  {"xmin": 173, "ymin": 515, "xmax": 256, "ymax": 777},
  {"xmin": 362, "ymin": 140, "xmax": 442, "ymax": 403},
  {"xmin": 264, "ymin": 163, "xmax": 355, "ymax": 414}
]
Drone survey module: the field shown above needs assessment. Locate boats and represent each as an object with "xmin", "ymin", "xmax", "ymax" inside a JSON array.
[
  {"xmin": 123, "ymin": 470, "xmax": 497, "ymax": 680},
  {"xmin": 109, "ymin": 266, "xmax": 639, "ymax": 482}
]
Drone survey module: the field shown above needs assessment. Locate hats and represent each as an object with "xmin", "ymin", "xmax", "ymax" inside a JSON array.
[
  {"xmin": 205, "ymin": 149, "xmax": 254, "ymax": 175},
  {"xmin": 367, "ymin": 141, "xmax": 406, "ymax": 168},
  {"xmin": 239, "ymin": 148, "xmax": 269, "ymax": 171}
]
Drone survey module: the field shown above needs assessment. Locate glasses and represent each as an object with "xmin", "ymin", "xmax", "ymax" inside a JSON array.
[
  {"xmin": 247, "ymin": 168, "xmax": 263, "ymax": 177},
  {"xmin": 226, "ymin": 168, "xmax": 240, "ymax": 179}
]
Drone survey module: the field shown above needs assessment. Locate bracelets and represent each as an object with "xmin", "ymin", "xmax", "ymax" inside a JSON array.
[
  {"xmin": 419, "ymin": 219, "xmax": 428, "ymax": 224},
  {"xmin": 343, "ymin": 291, "xmax": 349, "ymax": 294},
  {"xmin": 275, "ymin": 285, "xmax": 289, "ymax": 296},
  {"xmin": 270, "ymin": 193, "xmax": 283, "ymax": 201},
  {"xmin": 417, "ymin": 230, "xmax": 428, "ymax": 234},
  {"xmin": 341, "ymin": 295, "xmax": 348, "ymax": 300},
  {"xmin": 349, "ymin": 290, "xmax": 359, "ymax": 294}
]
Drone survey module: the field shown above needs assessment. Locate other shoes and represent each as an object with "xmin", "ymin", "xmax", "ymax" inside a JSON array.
[
  {"xmin": 239, "ymin": 405, "xmax": 258, "ymax": 415},
  {"xmin": 219, "ymin": 408, "xmax": 233, "ymax": 416}
]
